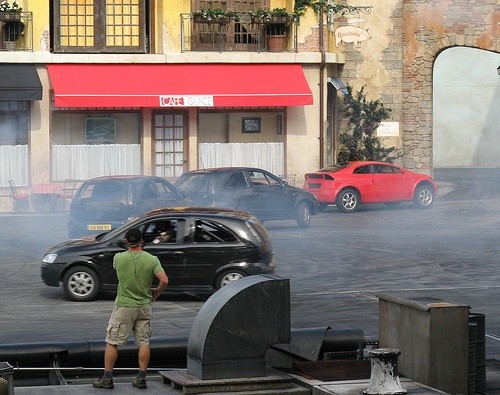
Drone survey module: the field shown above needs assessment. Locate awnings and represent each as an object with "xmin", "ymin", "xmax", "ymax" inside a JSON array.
[
  {"xmin": 0, "ymin": 63, "xmax": 42, "ymax": 100},
  {"xmin": 43, "ymin": 64, "xmax": 313, "ymax": 108}
]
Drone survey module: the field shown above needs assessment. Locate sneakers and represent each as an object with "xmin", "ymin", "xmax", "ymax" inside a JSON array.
[
  {"xmin": 132, "ymin": 378, "xmax": 146, "ymax": 389},
  {"xmin": 93, "ymin": 377, "xmax": 114, "ymax": 389}
]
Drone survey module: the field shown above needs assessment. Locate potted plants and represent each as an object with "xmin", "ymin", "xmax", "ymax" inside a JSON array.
[
  {"xmin": 263, "ymin": 24, "xmax": 291, "ymax": 51},
  {"xmin": 2, "ymin": 21, "xmax": 25, "ymax": 50}
]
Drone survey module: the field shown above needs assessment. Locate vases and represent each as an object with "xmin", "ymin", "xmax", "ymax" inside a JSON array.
[
  {"xmin": 195, "ymin": 15, "xmax": 230, "ymax": 23},
  {"xmin": 254, "ymin": 16, "xmax": 286, "ymax": 23},
  {"xmin": 5, "ymin": 13, "xmax": 20, "ymax": 19}
]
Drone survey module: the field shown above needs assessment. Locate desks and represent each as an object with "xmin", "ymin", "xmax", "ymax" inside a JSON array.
[{"xmin": 33, "ymin": 184, "xmax": 60, "ymax": 194}]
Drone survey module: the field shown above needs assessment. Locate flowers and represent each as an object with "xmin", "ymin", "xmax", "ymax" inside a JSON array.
[
  {"xmin": 0, "ymin": 1, "xmax": 22, "ymax": 13},
  {"xmin": 257, "ymin": 7, "xmax": 295, "ymax": 24},
  {"xmin": 192, "ymin": 8, "xmax": 240, "ymax": 25}
]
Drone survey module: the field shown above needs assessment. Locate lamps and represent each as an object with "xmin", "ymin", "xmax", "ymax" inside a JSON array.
[{"xmin": 497, "ymin": 66, "xmax": 500, "ymax": 75}]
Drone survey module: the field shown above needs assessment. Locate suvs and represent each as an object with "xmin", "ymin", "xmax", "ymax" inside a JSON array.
[{"xmin": 40, "ymin": 207, "xmax": 275, "ymax": 303}]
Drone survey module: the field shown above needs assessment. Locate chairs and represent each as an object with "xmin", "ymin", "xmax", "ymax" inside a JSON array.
[
  {"xmin": 9, "ymin": 180, "xmax": 28, "ymax": 214},
  {"xmin": 58, "ymin": 183, "xmax": 78, "ymax": 211}
]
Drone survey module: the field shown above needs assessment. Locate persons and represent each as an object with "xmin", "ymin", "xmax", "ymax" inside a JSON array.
[
  {"xmin": 93, "ymin": 228, "xmax": 168, "ymax": 389},
  {"xmin": 152, "ymin": 228, "xmax": 173, "ymax": 244}
]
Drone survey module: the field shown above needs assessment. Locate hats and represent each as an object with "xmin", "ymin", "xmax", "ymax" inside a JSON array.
[{"xmin": 126, "ymin": 228, "xmax": 142, "ymax": 243}]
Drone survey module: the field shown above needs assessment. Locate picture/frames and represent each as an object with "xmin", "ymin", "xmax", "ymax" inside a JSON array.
[
  {"xmin": 241, "ymin": 117, "xmax": 262, "ymax": 133},
  {"xmin": 83, "ymin": 118, "xmax": 119, "ymax": 144}
]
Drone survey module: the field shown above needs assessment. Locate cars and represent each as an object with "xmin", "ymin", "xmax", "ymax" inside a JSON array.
[
  {"xmin": 303, "ymin": 161, "xmax": 438, "ymax": 213},
  {"xmin": 67, "ymin": 175, "xmax": 195, "ymax": 241},
  {"xmin": 172, "ymin": 167, "xmax": 319, "ymax": 228}
]
